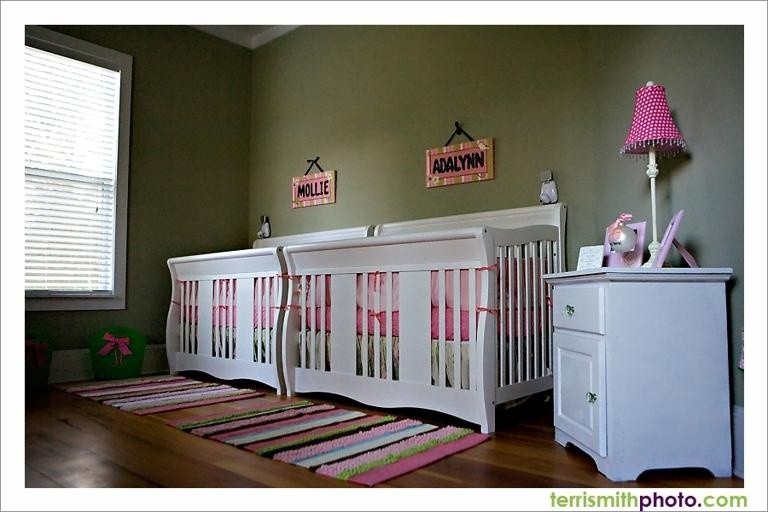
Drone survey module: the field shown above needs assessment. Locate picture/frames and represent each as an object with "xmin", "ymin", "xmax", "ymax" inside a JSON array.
[
  {"xmin": 425, "ymin": 137, "xmax": 494, "ymax": 188},
  {"xmin": 655, "ymin": 210, "xmax": 698, "ymax": 268},
  {"xmin": 603, "ymin": 220, "xmax": 646, "ymax": 268},
  {"xmin": 291, "ymin": 170, "xmax": 335, "ymax": 208}
]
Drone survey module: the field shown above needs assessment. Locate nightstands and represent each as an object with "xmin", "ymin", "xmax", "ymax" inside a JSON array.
[{"xmin": 540, "ymin": 266, "xmax": 735, "ymax": 482}]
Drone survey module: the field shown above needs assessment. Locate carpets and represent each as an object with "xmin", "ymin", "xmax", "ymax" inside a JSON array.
[
  {"xmin": 179, "ymin": 400, "xmax": 489, "ymax": 488},
  {"xmin": 62, "ymin": 374, "xmax": 264, "ymax": 415}
]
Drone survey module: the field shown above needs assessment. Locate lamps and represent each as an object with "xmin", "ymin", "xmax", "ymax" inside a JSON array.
[{"xmin": 619, "ymin": 80, "xmax": 690, "ymax": 268}]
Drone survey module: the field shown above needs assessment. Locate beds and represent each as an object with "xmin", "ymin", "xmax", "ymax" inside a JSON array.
[
  {"xmin": 165, "ymin": 223, "xmax": 375, "ymax": 396},
  {"xmin": 282, "ymin": 203, "xmax": 567, "ymax": 436}
]
